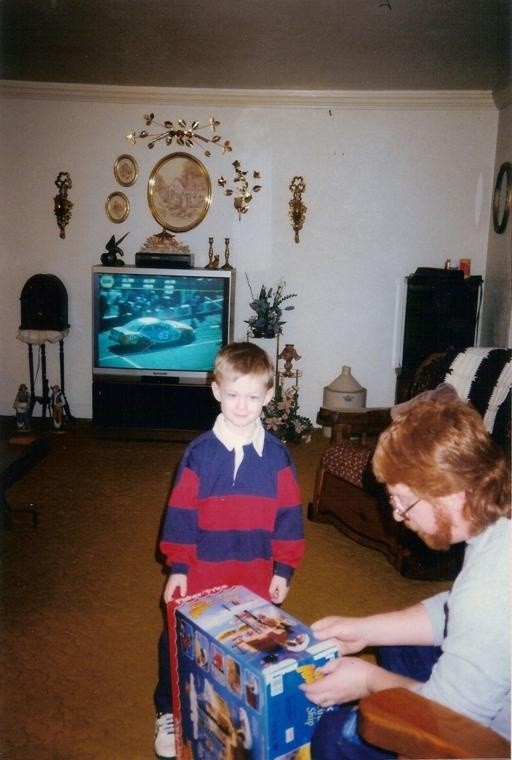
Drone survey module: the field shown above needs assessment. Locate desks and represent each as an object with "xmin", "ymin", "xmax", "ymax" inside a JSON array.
[
  {"xmin": 16, "ymin": 330, "xmax": 75, "ymax": 428},
  {"xmin": 0, "ymin": 433, "xmax": 50, "ymax": 535}
]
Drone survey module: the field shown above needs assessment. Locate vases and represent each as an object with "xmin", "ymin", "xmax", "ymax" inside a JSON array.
[
  {"xmin": 252, "ymin": 330, "xmax": 275, "ymax": 340},
  {"xmin": 275, "ymin": 433, "xmax": 291, "ymax": 445}
]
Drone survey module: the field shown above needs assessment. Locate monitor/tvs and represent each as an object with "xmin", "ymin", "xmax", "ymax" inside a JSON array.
[{"xmin": 92, "ymin": 265, "xmax": 236, "ymax": 386}]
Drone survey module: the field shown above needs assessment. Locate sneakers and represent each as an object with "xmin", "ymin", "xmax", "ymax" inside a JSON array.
[{"xmin": 154, "ymin": 713, "xmax": 176, "ymax": 759}]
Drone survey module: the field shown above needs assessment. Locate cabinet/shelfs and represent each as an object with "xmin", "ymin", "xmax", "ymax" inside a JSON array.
[
  {"xmin": 247, "ymin": 332, "xmax": 279, "ymax": 420},
  {"xmin": 90, "ymin": 376, "xmax": 220, "ymax": 442}
]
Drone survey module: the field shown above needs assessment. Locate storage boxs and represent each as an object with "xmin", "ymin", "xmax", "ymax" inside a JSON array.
[{"xmin": 167, "ymin": 583, "xmax": 340, "ymax": 759}]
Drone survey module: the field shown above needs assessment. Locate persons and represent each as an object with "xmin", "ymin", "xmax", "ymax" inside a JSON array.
[
  {"xmin": 297, "ymin": 383, "xmax": 511, "ymax": 760},
  {"xmin": 153, "ymin": 342, "xmax": 304, "ymax": 759}
]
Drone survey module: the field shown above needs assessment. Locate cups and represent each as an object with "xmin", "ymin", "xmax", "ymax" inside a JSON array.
[{"xmin": 460, "ymin": 259, "xmax": 471, "ymax": 279}]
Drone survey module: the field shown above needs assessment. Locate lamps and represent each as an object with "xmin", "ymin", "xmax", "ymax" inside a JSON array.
[{"xmin": 278, "ymin": 345, "xmax": 301, "ymax": 377}]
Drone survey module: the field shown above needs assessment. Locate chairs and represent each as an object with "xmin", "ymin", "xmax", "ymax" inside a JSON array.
[
  {"xmin": 308, "ymin": 347, "xmax": 512, "ymax": 580},
  {"xmin": 358, "ymin": 687, "xmax": 511, "ymax": 758}
]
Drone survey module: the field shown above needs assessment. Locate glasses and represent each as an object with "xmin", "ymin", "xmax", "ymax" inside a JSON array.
[{"xmin": 388, "ymin": 498, "xmax": 421, "ymax": 520}]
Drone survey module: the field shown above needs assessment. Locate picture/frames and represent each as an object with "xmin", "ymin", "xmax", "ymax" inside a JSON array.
[
  {"xmin": 105, "ymin": 191, "xmax": 130, "ymax": 224},
  {"xmin": 147, "ymin": 152, "xmax": 212, "ymax": 232},
  {"xmin": 492, "ymin": 162, "xmax": 512, "ymax": 233},
  {"xmin": 112, "ymin": 154, "xmax": 139, "ymax": 187}
]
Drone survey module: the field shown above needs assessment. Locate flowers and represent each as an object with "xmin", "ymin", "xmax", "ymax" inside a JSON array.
[
  {"xmin": 244, "ymin": 272, "xmax": 298, "ymax": 336},
  {"xmin": 260, "ymin": 387, "xmax": 314, "ymax": 446}
]
